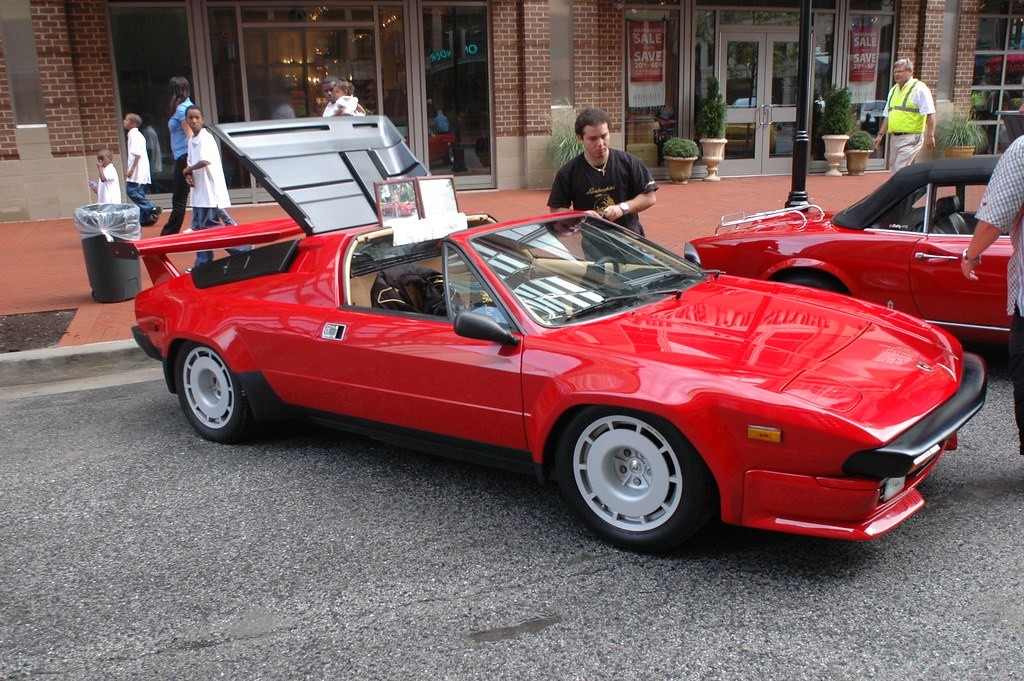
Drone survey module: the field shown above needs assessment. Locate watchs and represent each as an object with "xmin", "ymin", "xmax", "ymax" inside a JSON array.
[
  {"xmin": 963, "ymin": 246, "xmax": 981, "ymax": 265},
  {"xmin": 619, "ymin": 201, "xmax": 630, "ymax": 216}
]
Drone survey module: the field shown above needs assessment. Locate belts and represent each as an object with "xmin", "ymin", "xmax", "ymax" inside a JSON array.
[{"xmin": 894, "ymin": 133, "xmax": 907, "ymax": 136}]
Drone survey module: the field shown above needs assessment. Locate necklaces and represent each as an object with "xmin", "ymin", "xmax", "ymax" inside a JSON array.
[{"xmin": 584, "ymin": 151, "xmax": 609, "ymax": 175}]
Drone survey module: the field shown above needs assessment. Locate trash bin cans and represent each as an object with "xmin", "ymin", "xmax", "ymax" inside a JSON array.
[{"xmin": 76, "ymin": 203, "xmax": 143, "ymax": 302}]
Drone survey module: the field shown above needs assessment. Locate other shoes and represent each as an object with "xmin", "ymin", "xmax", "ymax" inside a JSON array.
[
  {"xmin": 185, "ymin": 268, "xmax": 192, "ymax": 273},
  {"xmin": 183, "ymin": 228, "xmax": 194, "ymax": 233},
  {"xmin": 151, "ymin": 206, "xmax": 162, "ymax": 223}
]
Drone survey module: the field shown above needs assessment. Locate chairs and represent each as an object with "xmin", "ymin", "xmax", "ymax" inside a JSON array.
[
  {"xmin": 369, "ymin": 262, "xmax": 465, "ymax": 317},
  {"xmin": 897, "ymin": 205, "xmax": 945, "ymax": 234},
  {"xmin": 935, "ymin": 195, "xmax": 972, "ymax": 235}
]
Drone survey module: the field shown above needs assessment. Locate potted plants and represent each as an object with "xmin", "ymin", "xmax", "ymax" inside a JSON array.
[
  {"xmin": 969, "ymin": 91, "xmax": 986, "ymax": 111},
  {"xmin": 820, "ymin": 84, "xmax": 855, "ymax": 177},
  {"xmin": 1008, "ymin": 97, "xmax": 1022, "ymax": 110},
  {"xmin": 845, "ymin": 130, "xmax": 875, "ymax": 176},
  {"xmin": 934, "ymin": 114, "xmax": 988, "ymax": 158},
  {"xmin": 662, "ymin": 137, "xmax": 701, "ymax": 185},
  {"xmin": 695, "ymin": 76, "xmax": 729, "ymax": 183}
]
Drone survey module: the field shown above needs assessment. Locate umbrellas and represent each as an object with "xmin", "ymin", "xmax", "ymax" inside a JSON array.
[
  {"xmin": 985, "ymin": 55, "xmax": 1024, "ymax": 76},
  {"xmin": 771, "ymin": 52, "xmax": 827, "ymax": 77}
]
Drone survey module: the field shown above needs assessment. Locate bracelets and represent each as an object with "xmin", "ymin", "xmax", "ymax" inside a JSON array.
[{"xmin": 876, "ymin": 131, "xmax": 884, "ymax": 136}]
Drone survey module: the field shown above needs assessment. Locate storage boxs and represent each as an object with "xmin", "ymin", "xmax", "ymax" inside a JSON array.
[{"xmin": 627, "ymin": 116, "xmax": 655, "ymax": 144}]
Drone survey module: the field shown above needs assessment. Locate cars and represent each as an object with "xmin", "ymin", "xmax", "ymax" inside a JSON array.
[
  {"xmin": 852, "ymin": 99, "xmax": 887, "ymax": 128},
  {"xmin": 383, "ymin": 90, "xmax": 458, "ymax": 167},
  {"xmin": 107, "ymin": 115, "xmax": 989, "ymax": 555},
  {"xmin": 684, "ymin": 156, "xmax": 1016, "ymax": 362},
  {"xmin": 723, "ymin": 98, "xmax": 778, "ymax": 155}
]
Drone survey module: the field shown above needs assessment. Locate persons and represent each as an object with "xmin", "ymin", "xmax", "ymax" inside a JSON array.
[
  {"xmin": 272, "ymin": 83, "xmax": 297, "ymax": 119},
  {"xmin": 137, "ymin": 114, "xmax": 167, "ymax": 194},
  {"xmin": 816, "ymin": 95, "xmax": 826, "ymax": 115},
  {"xmin": 873, "ymin": 59, "xmax": 936, "ymax": 177},
  {"xmin": 961, "ymin": 91, "xmax": 1024, "ymax": 456},
  {"xmin": 320, "ymin": 74, "xmax": 367, "ymax": 118},
  {"xmin": 549, "ymin": 108, "xmax": 659, "ymax": 265},
  {"xmin": 435, "ymin": 110, "xmax": 451, "ymax": 135},
  {"xmin": 87, "ymin": 75, "xmax": 255, "ymax": 275},
  {"xmin": 390, "ymin": 190, "xmax": 400, "ymax": 218}
]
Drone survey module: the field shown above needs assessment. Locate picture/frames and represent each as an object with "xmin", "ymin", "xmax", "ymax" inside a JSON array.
[
  {"xmin": 416, "ymin": 174, "xmax": 460, "ymax": 219},
  {"xmin": 372, "ymin": 176, "xmax": 422, "ymax": 229}
]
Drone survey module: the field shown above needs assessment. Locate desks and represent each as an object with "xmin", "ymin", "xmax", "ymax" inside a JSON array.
[{"xmin": 725, "ymin": 124, "xmax": 776, "ymax": 155}]
